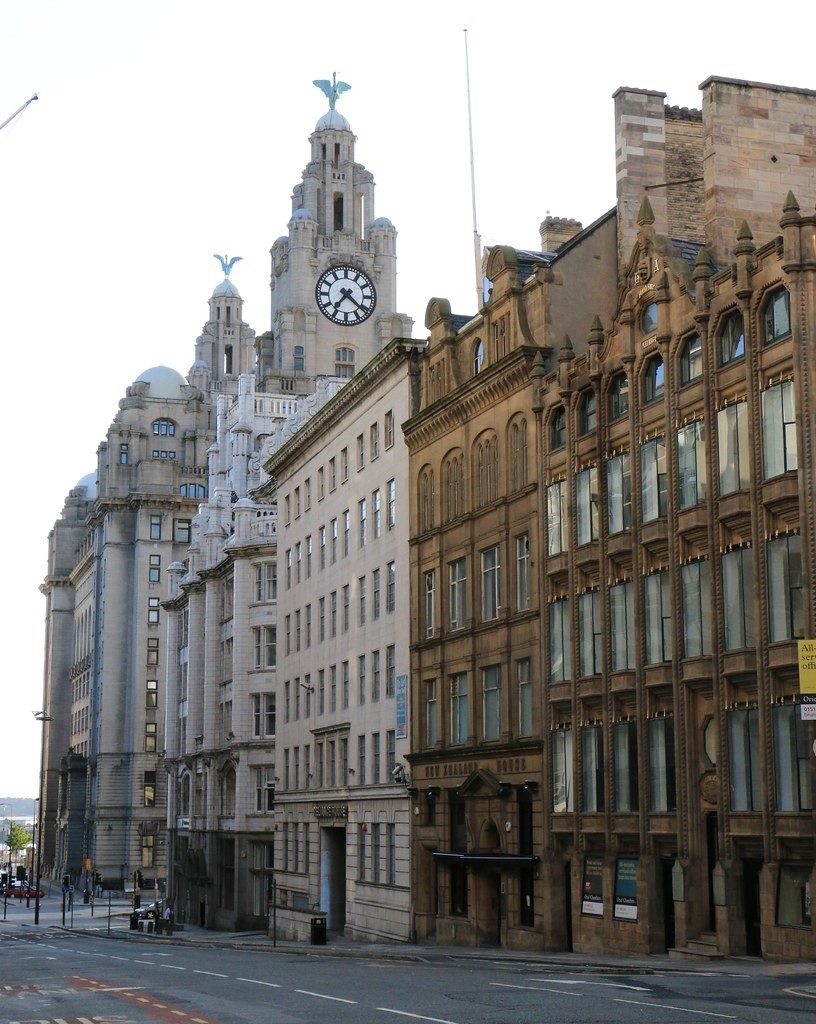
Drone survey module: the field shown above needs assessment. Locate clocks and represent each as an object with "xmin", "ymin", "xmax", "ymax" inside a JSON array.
[{"xmin": 315, "ymin": 265, "xmax": 378, "ymax": 326}]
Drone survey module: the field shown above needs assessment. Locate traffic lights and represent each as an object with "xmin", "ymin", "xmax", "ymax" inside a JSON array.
[
  {"xmin": 2, "ymin": 874, "xmax": 7, "ymax": 887},
  {"xmin": 63, "ymin": 875, "xmax": 70, "ymax": 892},
  {"xmin": 95, "ymin": 872, "xmax": 103, "ymax": 886}
]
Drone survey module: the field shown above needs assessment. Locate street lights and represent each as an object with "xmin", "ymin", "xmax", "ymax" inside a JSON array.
[{"xmin": 35, "ymin": 704, "xmax": 54, "ymax": 924}]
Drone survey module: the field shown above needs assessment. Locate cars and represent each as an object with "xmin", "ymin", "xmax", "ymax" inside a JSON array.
[
  {"xmin": 135, "ymin": 900, "xmax": 162, "ymax": 919},
  {"xmin": 3, "ymin": 876, "xmax": 44, "ymax": 898}
]
